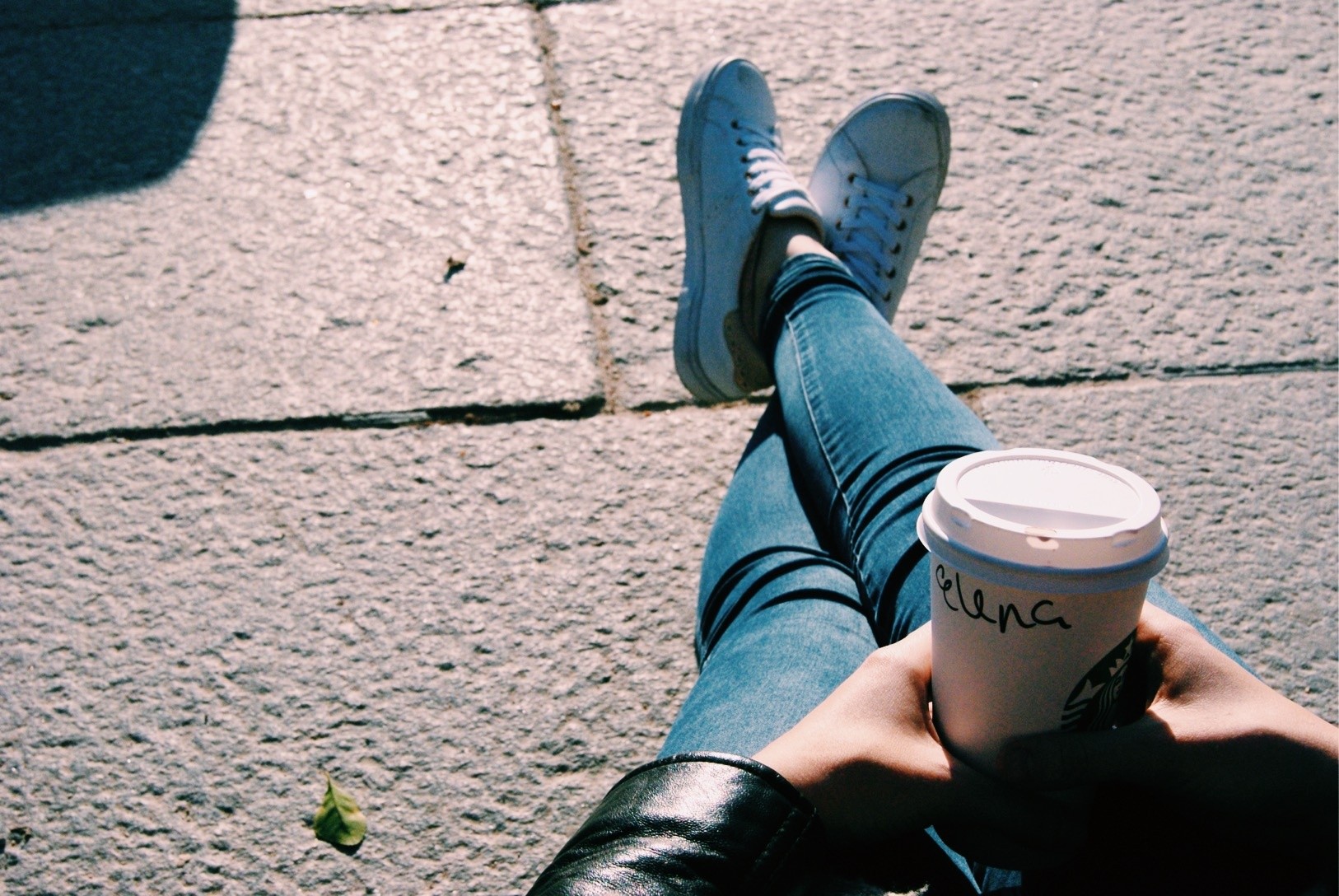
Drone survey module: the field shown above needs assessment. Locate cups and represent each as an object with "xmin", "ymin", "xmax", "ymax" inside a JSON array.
[{"xmin": 915, "ymin": 447, "xmax": 1170, "ymax": 781}]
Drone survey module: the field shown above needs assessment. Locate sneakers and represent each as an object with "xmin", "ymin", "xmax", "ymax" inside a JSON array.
[
  {"xmin": 802, "ymin": 89, "xmax": 950, "ymax": 326},
  {"xmin": 673, "ymin": 57, "xmax": 827, "ymax": 405}
]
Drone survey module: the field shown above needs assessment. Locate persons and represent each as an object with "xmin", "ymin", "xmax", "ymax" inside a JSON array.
[{"xmin": 528, "ymin": 53, "xmax": 1339, "ymax": 896}]
